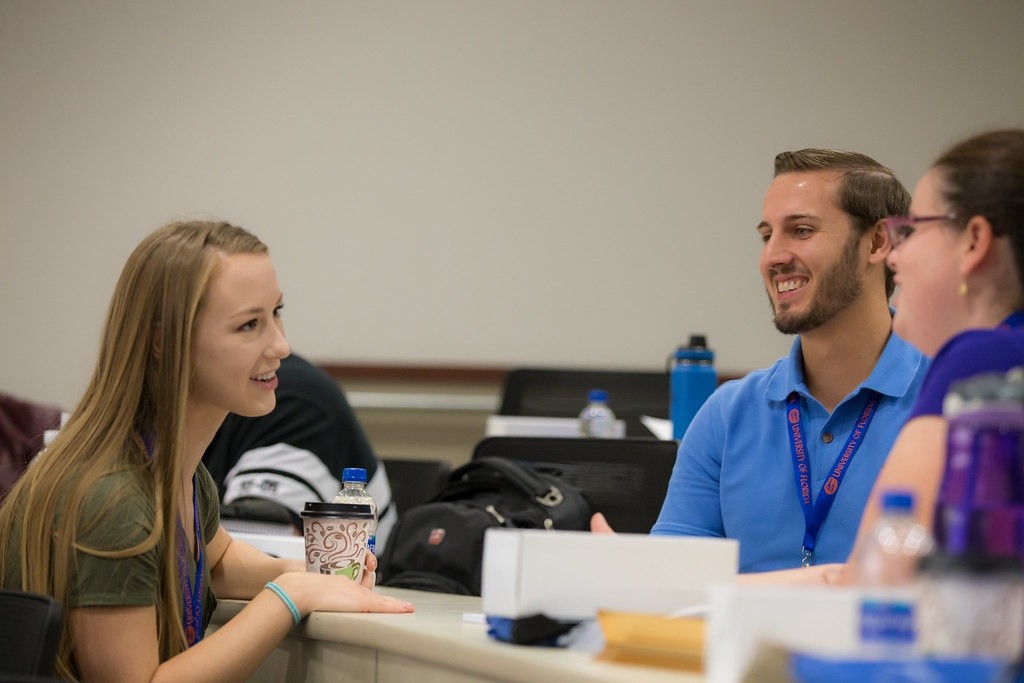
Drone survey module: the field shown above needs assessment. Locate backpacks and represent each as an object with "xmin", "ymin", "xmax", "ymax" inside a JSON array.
[{"xmin": 428, "ymin": 455, "xmax": 591, "ymax": 540}]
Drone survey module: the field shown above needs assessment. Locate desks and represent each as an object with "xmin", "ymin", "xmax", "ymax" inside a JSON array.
[{"xmin": 198, "ymin": 584, "xmax": 709, "ymax": 683}]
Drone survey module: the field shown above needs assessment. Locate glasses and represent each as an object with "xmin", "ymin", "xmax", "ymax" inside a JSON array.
[{"xmin": 886, "ymin": 215, "xmax": 959, "ymax": 248}]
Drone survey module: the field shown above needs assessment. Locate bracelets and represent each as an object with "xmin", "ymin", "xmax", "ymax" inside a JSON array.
[{"xmin": 265, "ymin": 583, "xmax": 302, "ymax": 630}]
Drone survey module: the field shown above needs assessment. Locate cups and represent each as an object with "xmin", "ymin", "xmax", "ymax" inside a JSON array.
[{"xmin": 300, "ymin": 502, "xmax": 375, "ymax": 586}]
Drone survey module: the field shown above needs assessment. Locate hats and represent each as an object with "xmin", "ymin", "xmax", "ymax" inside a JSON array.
[
  {"xmin": 222, "ymin": 443, "xmax": 341, "ymax": 524},
  {"xmin": 377, "ymin": 505, "xmax": 495, "ymax": 595}
]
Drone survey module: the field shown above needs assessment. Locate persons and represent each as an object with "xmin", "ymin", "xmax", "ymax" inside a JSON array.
[
  {"xmin": 0, "ymin": 221, "xmax": 415, "ymax": 683},
  {"xmin": 590, "ymin": 127, "xmax": 1024, "ymax": 591}
]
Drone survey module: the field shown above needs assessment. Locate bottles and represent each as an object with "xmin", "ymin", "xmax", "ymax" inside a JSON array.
[
  {"xmin": 579, "ymin": 389, "xmax": 617, "ymax": 438},
  {"xmin": 332, "ymin": 468, "xmax": 376, "ymax": 586},
  {"xmin": 858, "ymin": 493, "xmax": 914, "ymax": 655},
  {"xmin": 668, "ymin": 335, "xmax": 717, "ymax": 440}
]
